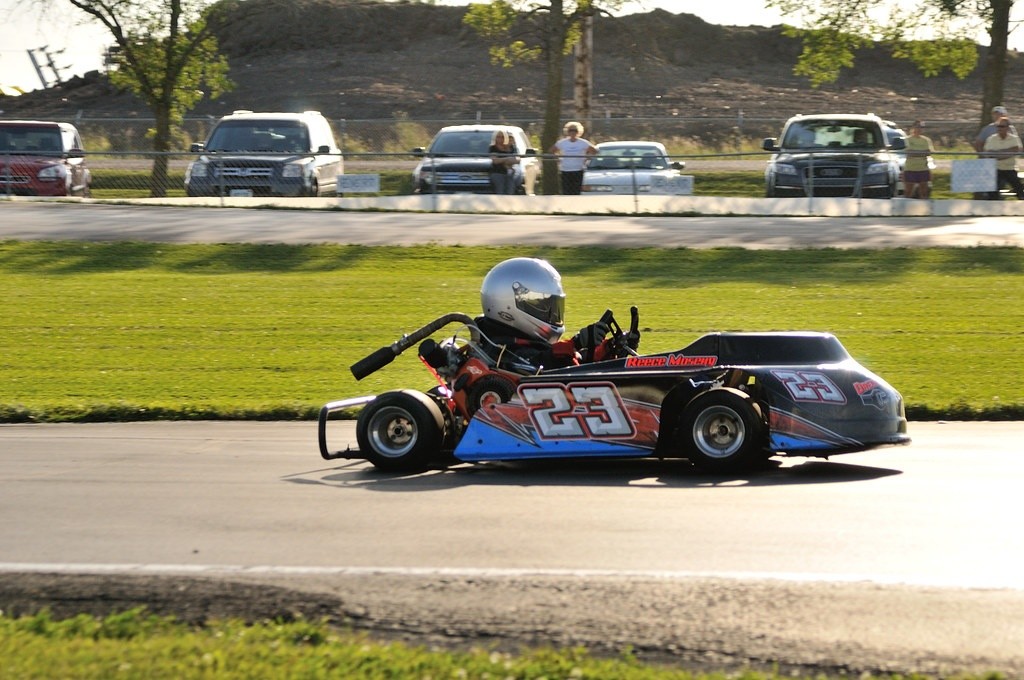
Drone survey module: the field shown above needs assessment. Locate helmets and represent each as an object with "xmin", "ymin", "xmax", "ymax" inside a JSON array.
[{"xmin": 481, "ymin": 257, "xmax": 565, "ymax": 344}]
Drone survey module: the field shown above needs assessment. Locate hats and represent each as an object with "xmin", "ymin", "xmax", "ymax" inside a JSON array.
[
  {"xmin": 909, "ymin": 120, "xmax": 926, "ymax": 128},
  {"xmin": 992, "ymin": 106, "xmax": 1007, "ymax": 115}
]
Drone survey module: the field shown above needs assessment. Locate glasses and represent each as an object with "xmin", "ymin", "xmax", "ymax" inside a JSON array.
[
  {"xmin": 568, "ymin": 129, "xmax": 577, "ymax": 132},
  {"xmin": 994, "ymin": 125, "xmax": 1008, "ymax": 128}
]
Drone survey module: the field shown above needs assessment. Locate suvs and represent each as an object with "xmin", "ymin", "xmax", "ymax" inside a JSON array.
[
  {"xmin": 760, "ymin": 112, "xmax": 907, "ymax": 201},
  {"xmin": 412, "ymin": 125, "xmax": 539, "ymax": 195},
  {"xmin": 184, "ymin": 108, "xmax": 344, "ymax": 198},
  {"xmin": 1, "ymin": 121, "xmax": 93, "ymax": 203}
]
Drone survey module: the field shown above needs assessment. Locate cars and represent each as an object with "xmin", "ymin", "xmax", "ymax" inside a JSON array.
[
  {"xmin": 580, "ymin": 138, "xmax": 696, "ymax": 196},
  {"xmin": 816, "ymin": 119, "xmax": 939, "ymax": 173}
]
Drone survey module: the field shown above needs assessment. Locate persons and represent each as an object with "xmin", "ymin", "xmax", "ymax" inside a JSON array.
[
  {"xmin": 983, "ymin": 117, "xmax": 1024, "ymax": 200},
  {"xmin": 488, "ymin": 129, "xmax": 520, "ymax": 195},
  {"xmin": 903, "ymin": 121, "xmax": 934, "ymax": 199},
  {"xmin": 474, "ymin": 257, "xmax": 640, "ymax": 370},
  {"xmin": 974, "ymin": 106, "xmax": 1018, "ymax": 200},
  {"xmin": 547, "ymin": 121, "xmax": 599, "ymax": 194}
]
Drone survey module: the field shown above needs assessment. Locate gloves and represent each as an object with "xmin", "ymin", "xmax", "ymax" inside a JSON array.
[
  {"xmin": 572, "ymin": 322, "xmax": 609, "ymax": 350},
  {"xmin": 610, "ymin": 330, "xmax": 639, "ymax": 354}
]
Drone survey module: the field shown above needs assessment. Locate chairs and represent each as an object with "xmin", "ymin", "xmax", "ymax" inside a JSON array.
[
  {"xmin": 640, "ymin": 153, "xmax": 659, "ymax": 166},
  {"xmin": 602, "ymin": 159, "xmax": 620, "ymax": 167},
  {"xmin": 800, "ymin": 129, "xmax": 823, "ymax": 147},
  {"xmin": 847, "ymin": 129, "xmax": 874, "ymax": 147}
]
